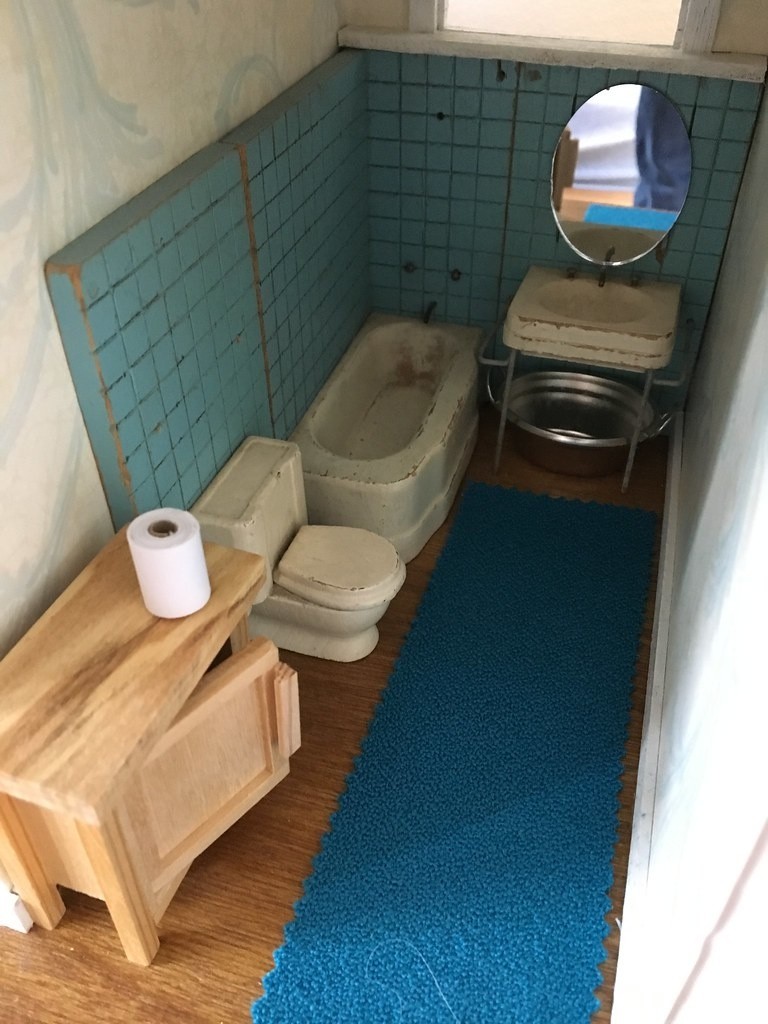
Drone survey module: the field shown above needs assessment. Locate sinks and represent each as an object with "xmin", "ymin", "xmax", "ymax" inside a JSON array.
[{"xmin": 500, "ymin": 263, "xmax": 685, "ymax": 376}]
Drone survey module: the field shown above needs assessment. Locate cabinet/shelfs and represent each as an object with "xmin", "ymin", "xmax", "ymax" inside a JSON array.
[{"xmin": 0, "ymin": 522, "xmax": 304, "ymax": 970}]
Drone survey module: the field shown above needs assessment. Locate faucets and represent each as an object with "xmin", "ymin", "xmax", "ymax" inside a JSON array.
[
  {"xmin": 603, "ymin": 245, "xmax": 616, "ymax": 262},
  {"xmin": 597, "ymin": 265, "xmax": 607, "ymax": 288}
]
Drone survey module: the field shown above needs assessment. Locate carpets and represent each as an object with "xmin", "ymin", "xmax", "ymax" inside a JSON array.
[{"xmin": 249, "ymin": 481, "xmax": 659, "ymax": 1024}]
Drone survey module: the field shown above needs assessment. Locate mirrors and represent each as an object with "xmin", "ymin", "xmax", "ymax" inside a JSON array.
[{"xmin": 550, "ymin": 81, "xmax": 694, "ymax": 267}]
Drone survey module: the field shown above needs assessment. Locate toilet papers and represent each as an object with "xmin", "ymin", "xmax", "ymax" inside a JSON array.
[{"xmin": 125, "ymin": 506, "xmax": 212, "ymax": 621}]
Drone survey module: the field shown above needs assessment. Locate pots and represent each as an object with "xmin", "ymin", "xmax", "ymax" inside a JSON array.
[{"xmin": 482, "ymin": 363, "xmax": 675, "ymax": 479}]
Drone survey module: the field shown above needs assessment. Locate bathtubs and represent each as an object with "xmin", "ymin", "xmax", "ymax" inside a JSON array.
[{"xmin": 284, "ymin": 310, "xmax": 485, "ymax": 567}]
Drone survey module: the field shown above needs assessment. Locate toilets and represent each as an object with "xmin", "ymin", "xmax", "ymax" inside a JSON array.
[{"xmin": 184, "ymin": 434, "xmax": 408, "ymax": 666}]
